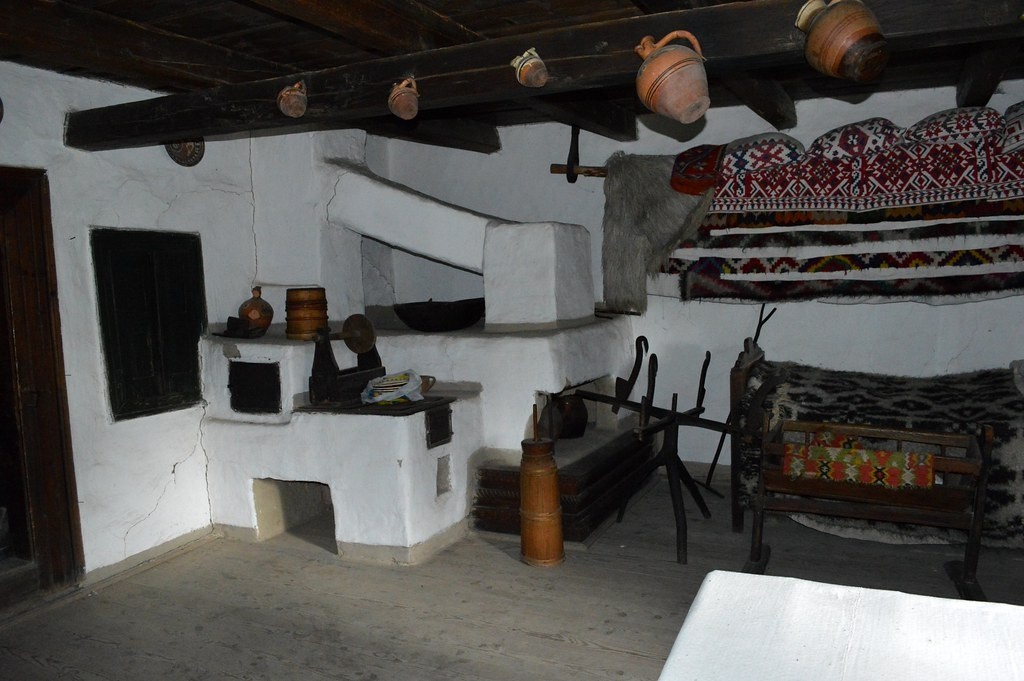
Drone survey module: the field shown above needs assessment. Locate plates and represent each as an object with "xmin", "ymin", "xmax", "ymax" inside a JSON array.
[{"xmin": 373, "ymin": 381, "xmax": 408, "ymax": 392}]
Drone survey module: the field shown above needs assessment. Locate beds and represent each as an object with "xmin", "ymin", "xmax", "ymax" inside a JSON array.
[
  {"xmin": 730, "ymin": 338, "xmax": 1024, "ymax": 552},
  {"xmin": 742, "ymin": 414, "xmax": 993, "ymax": 602}
]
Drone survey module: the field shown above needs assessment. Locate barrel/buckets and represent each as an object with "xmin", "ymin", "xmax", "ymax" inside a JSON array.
[{"xmin": 286, "ymin": 288, "xmax": 330, "ymax": 342}]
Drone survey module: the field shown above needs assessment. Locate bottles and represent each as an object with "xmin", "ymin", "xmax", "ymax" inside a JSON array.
[{"xmin": 239, "ymin": 286, "xmax": 274, "ymax": 337}]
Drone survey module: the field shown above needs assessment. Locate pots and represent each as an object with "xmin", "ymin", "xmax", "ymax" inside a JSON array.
[{"xmin": 392, "ymin": 299, "xmax": 484, "ymax": 332}]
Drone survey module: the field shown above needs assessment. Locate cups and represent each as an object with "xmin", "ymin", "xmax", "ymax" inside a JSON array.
[{"xmin": 420, "ymin": 375, "xmax": 437, "ymax": 393}]
